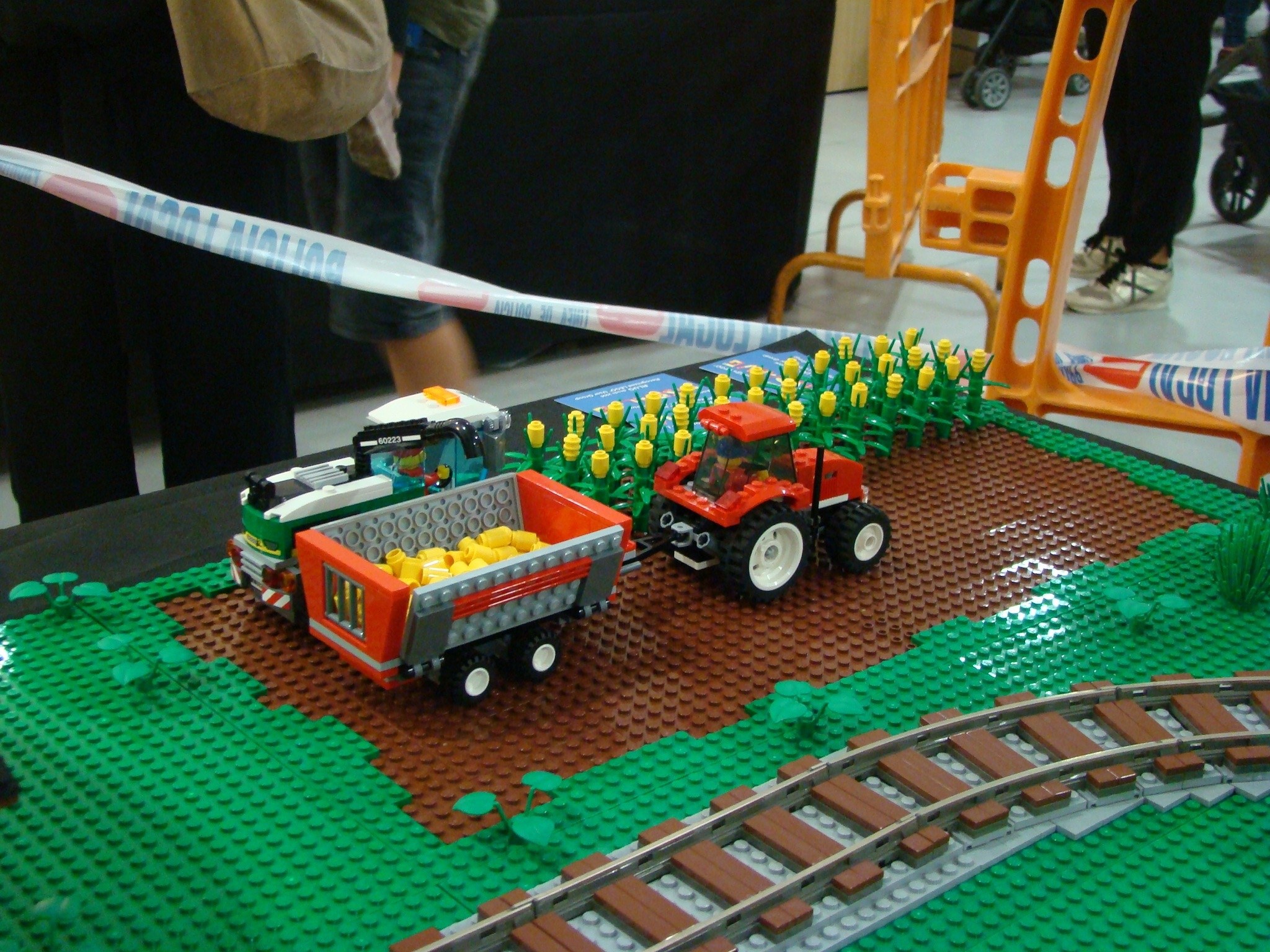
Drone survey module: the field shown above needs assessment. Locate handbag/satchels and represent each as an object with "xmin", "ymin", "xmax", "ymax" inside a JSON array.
[{"xmin": 165, "ymin": 0, "xmax": 394, "ymax": 140}]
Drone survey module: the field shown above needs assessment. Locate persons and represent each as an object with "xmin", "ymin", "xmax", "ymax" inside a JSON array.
[
  {"xmin": 326, "ymin": 0, "xmax": 500, "ymax": 399},
  {"xmin": 1, "ymin": 1, "xmax": 315, "ymax": 525},
  {"xmin": 1065, "ymin": 1, "xmax": 1223, "ymax": 315}
]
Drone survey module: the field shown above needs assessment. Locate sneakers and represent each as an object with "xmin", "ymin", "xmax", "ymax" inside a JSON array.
[
  {"xmin": 1064, "ymin": 247, "xmax": 1175, "ymax": 315},
  {"xmin": 1070, "ymin": 234, "xmax": 1127, "ymax": 281}
]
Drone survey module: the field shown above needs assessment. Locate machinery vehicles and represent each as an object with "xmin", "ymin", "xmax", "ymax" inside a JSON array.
[
  {"xmin": 295, "ymin": 399, "xmax": 892, "ymax": 708},
  {"xmin": 226, "ymin": 386, "xmax": 514, "ymax": 627}
]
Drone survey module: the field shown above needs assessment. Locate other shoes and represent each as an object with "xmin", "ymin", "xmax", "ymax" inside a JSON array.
[{"xmin": 344, "ymin": 81, "xmax": 402, "ymax": 181}]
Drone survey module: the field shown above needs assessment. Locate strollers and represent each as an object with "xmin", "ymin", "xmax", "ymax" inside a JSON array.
[{"xmin": 956, "ymin": 0, "xmax": 1101, "ymax": 111}]
